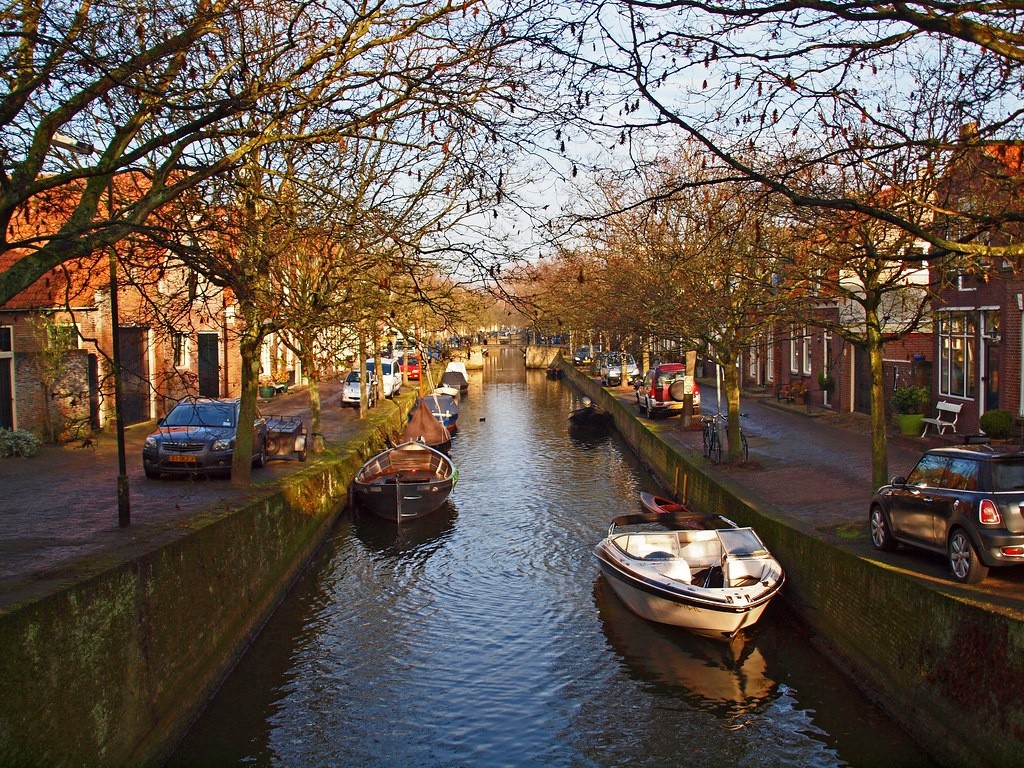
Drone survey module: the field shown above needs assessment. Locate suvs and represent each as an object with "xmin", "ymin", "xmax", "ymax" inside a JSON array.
[
  {"xmin": 869, "ymin": 439, "xmax": 1023, "ymax": 585},
  {"xmin": 142, "ymin": 396, "xmax": 269, "ymax": 479},
  {"xmin": 636, "ymin": 364, "xmax": 700, "ymax": 419}
]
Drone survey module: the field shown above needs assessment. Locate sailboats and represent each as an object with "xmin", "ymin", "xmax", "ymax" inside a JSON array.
[{"xmin": 388, "ymin": 317, "xmax": 452, "ymax": 457}]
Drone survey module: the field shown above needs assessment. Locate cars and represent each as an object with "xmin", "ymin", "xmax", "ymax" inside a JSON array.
[
  {"xmin": 359, "ymin": 357, "xmax": 402, "ymax": 399},
  {"xmin": 340, "ymin": 370, "xmax": 378, "ymax": 408},
  {"xmin": 396, "ymin": 354, "xmax": 420, "ymax": 380},
  {"xmin": 590, "ymin": 352, "xmax": 640, "ymax": 386},
  {"xmin": 572, "ymin": 346, "xmax": 598, "ymax": 366},
  {"xmin": 428, "ymin": 341, "xmax": 443, "ymax": 363},
  {"xmin": 447, "ymin": 330, "xmax": 510, "ymax": 347}
]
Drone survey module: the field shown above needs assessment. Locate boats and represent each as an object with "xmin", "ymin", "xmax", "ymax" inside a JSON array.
[
  {"xmin": 354, "ymin": 437, "xmax": 458, "ymax": 526},
  {"xmin": 568, "ymin": 397, "xmax": 614, "ymax": 431},
  {"xmin": 407, "ymin": 393, "xmax": 459, "ymax": 431},
  {"xmin": 591, "ymin": 509, "xmax": 786, "ymax": 641},
  {"xmin": 433, "ymin": 360, "xmax": 468, "ymax": 405},
  {"xmin": 639, "ymin": 491, "xmax": 704, "ymax": 530},
  {"xmin": 546, "ymin": 367, "xmax": 563, "ymax": 377}
]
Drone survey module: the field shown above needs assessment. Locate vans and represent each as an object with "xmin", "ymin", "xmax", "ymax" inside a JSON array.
[{"xmin": 394, "ymin": 339, "xmax": 417, "ymax": 357}]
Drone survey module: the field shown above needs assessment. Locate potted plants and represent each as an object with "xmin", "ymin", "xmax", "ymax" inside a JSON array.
[
  {"xmin": 889, "ymin": 385, "xmax": 930, "ymax": 435},
  {"xmin": 300, "ymin": 371, "xmax": 309, "ymax": 386},
  {"xmin": 270, "ymin": 366, "xmax": 290, "ymax": 393},
  {"xmin": 259, "ymin": 375, "xmax": 274, "ymax": 398},
  {"xmin": 978, "ymin": 409, "xmax": 1017, "ymax": 445},
  {"xmin": 332, "ymin": 360, "xmax": 346, "ymax": 371}
]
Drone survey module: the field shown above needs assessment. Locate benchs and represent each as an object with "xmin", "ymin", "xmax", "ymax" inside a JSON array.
[{"xmin": 919, "ymin": 400, "xmax": 964, "ymax": 439}]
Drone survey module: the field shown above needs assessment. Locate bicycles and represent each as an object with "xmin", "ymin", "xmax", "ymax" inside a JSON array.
[{"xmin": 700, "ymin": 413, "xmax": 748, "ymax": 466}]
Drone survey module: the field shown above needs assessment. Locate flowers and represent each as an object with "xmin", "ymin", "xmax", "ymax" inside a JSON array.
[{"xmin": 779, "ymin": 380, "xmax": 808, "ymax": 398}]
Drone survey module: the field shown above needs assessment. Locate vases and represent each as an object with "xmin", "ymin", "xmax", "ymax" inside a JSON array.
[{"xmin": 793, "ymin": 393, "xmax": 807, "ymax": 405}]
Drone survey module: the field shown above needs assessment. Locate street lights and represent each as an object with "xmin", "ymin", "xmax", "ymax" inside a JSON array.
[{"xmin": 51, "ymin": 133, "xmax": 131, "ymax": 528}]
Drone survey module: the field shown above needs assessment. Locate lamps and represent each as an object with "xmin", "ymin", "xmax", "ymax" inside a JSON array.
[{"xmin": 989, "ymin": 325, "xmax": 1001, "ymax": 342}]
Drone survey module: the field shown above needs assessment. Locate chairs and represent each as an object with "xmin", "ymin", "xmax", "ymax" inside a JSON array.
[{"xmin": 351, "ymin": 374, "xmax": 359, "ymax": 381}]
